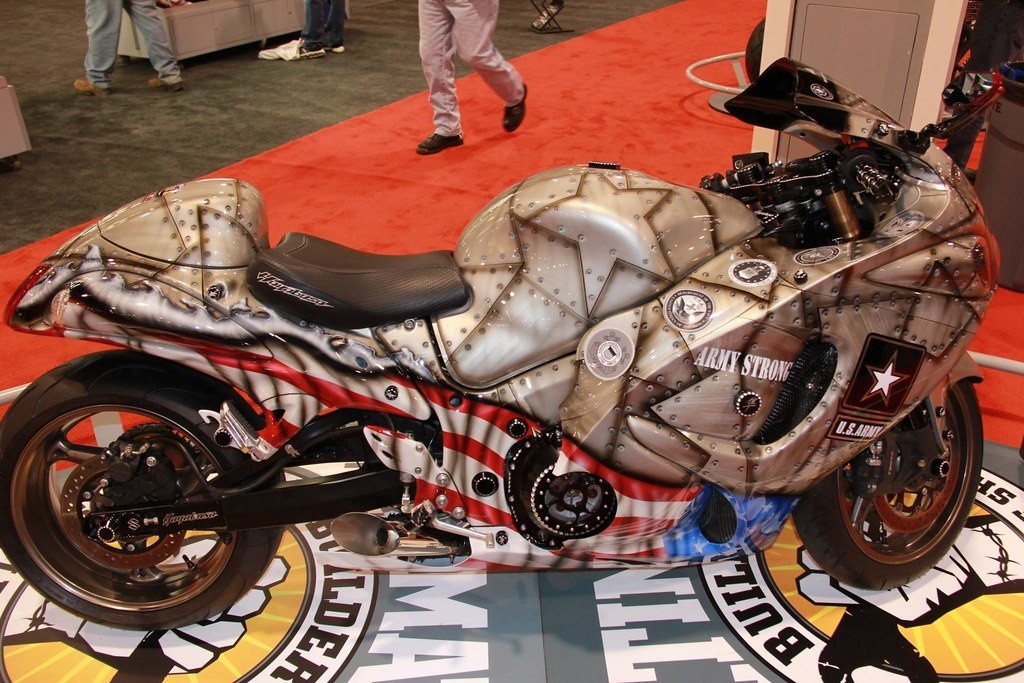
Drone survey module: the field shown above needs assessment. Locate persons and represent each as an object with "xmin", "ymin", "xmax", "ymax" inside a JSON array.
[
  {"xmin": 73, "ymin": 0, "xmax": 185, "ymax": 97},
  {"xmin": 293, "ymin": 0, "xmax": 345, "ymax": 60},
  {"xmin": 417, "ymin": 1, "xmax": 527, "ymax": 156}
]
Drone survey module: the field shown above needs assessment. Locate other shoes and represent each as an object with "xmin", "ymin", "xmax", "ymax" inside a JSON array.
[
  {"xmin": 323, "ymin": 38, "xmax": 345, "ymax": 52},
  {"xmin": 299, "ymin": 42, "xmax": 325, "ymax": 60},
  {"xmin": 416, "ymin": 131, "xmax": 464, "ymax": 154},
  {"xmin": 502, "ymin": 82, "xmax": 527, "ymax": 132}
]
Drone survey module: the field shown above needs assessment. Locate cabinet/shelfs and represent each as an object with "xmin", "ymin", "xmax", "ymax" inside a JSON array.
[{"xmin": 116, "ymin": 0, "xmax": 350, "ymax": 62}]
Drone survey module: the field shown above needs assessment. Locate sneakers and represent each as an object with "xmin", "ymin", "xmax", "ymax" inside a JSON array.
[
  {"xmin": 73, "ymin": 79, "xmax": 112, "ymax": 96},
  {"xmin": 148, "ymin": 78, "xmax": 184, "ymax": 91}
]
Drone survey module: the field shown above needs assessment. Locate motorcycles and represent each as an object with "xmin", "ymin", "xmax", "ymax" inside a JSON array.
[{"xmin": 0, "ymin": 57, "xmax": 1005, "ymax": 630}]
